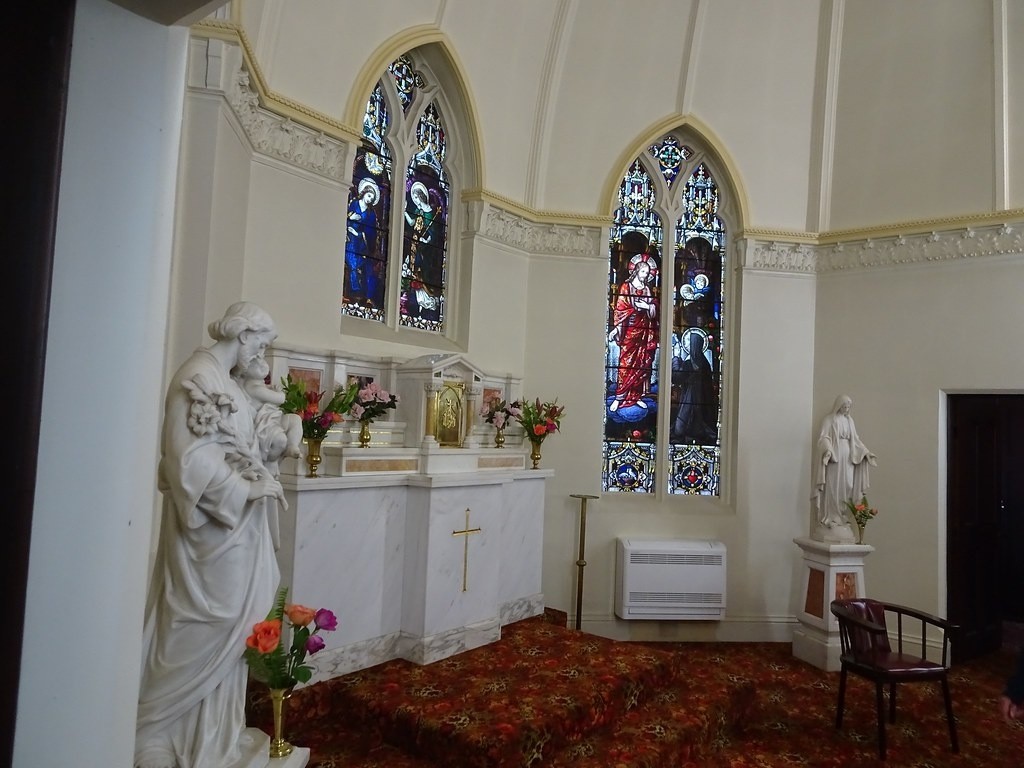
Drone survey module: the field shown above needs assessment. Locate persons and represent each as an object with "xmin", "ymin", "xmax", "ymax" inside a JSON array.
[
  {"xmin": 810, "ymin": 394, "xmax": 878, "ymax": 528},
  {"xmin": 136, "ymin": 302, "xmax": 305, "ymax": 768},
  {"xmin": 240, "ymin": 70, "xmax": 325, "ymax": 169}
]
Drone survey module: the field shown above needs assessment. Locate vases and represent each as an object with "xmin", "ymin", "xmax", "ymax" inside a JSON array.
[
  {"xmin": 304, "ymin": 438, "xmax": 324, "ymax": 478},
  {"xmin": 360, "ymin": 422, "xmax": 371, "ymax": 448},
  {"xmin": 495, "ymin": 425, "xmax": 505, "ymax": 448},
  {"xmin": 531, "ymin": 441, "xmax": 541, "ymax": 470},
  {"xmin": 857, "ymin": 523, "xmax": 867, "ymax": 546},
  {"xmin": 269, "ymin": 686, "xmax": 293, "ymax": 758}
]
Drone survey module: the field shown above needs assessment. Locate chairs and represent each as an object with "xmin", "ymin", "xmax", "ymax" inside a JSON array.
[{"xmin": 830, "ymin": 596, "xmax": 958, "ymax": 764}]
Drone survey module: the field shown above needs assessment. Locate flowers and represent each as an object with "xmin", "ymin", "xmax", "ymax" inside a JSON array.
[
  {"xmin": 241, "ymin": 585, "xmax": 336, "ymax": 697},
  {"xmin": 479, "ymin": 392, "xmax": 522, "ymax": 429},
  {"xmin": 844, "ymin": 497, "xmax": 878, "ymax": 526},
  {"xmin": 348, "ymin": 380, "xmax": 399, "ymax": 423},
  {"xmin": 510, "ymin": 396, "xmax": 566, "ymax": 443},
  {"xmin": 275, "ymin": 371, "xmax": 358, "ymax": 440}
]
